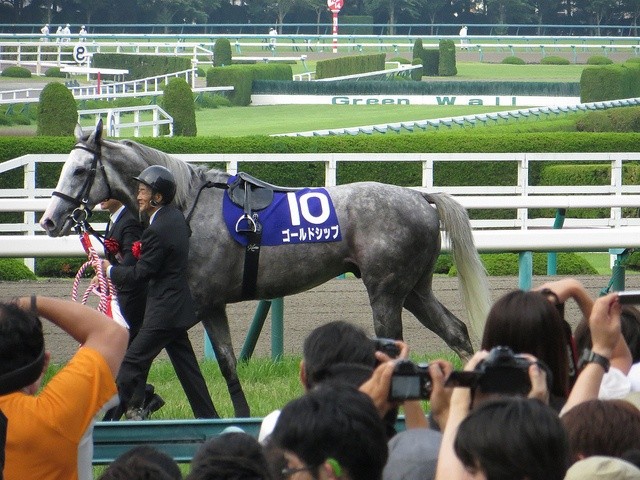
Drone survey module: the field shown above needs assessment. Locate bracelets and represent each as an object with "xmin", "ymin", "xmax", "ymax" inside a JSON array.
[
  {"xmin": 587, "ymin": 352, "xmax": 610, "ymax": 372},
  {"xmin": 31, "ymin": 296, "xmax": 37, "ymax": 311}
]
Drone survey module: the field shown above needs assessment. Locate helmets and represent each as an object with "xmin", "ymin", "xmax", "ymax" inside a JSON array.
[{"xmin": 131, "ymin": 165, "xmax": 176, "ymax": 204}]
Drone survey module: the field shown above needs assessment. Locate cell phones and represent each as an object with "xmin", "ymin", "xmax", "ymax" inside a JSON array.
[{"xmin": 619, "ymin": 292, "xmax": 640, "ymax": 306}]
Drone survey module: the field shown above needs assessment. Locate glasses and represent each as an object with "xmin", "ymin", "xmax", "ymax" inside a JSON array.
[{"xmin": 280, "ymin": 456, "xmax": 343, "ymax": 478}]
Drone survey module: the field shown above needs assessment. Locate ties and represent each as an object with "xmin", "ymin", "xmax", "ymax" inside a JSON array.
[{"xmin": 109, "ymin": 220, "xmax": 113, "ymax": 230}]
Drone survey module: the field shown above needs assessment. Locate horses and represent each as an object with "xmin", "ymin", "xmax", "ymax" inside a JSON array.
[{"xmin": 38, "ymin": 118, "xmax": 493, "ymax": 421}]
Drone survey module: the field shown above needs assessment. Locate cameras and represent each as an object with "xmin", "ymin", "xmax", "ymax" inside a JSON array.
[
  {"xmin": 390, "ymin": 359, "xmax": 432, "ymax": 401},
  {"xmin": 445, "ymin": 346, "xmax": 532, "ymax": 409},
  {"xmin": 373, "ymin": 339, "xmax": 399, "ymax": 369}
]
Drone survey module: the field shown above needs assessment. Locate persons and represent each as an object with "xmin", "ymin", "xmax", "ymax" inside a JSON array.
[
  {"xmin": 435, "ymin": 349, "xmax": 550, "ymax": 480},
  {"xmin": 186, "ymin": 432, "xmax": 271, "ymax": 479},
  {"xmin": 557, "ymin": 291, "xmax": 640, "ymax": 420},
  {"xmin": 259, "ymin": 321, "xmax": 410, "ymax": 446},
  {"xmin": 358, "ymin": 360, "xmax": 452, "ymax": 480},
  {"xmin": 100, "ymin": 199, "xmax": 145, "ymax": 350},
  {"xmin": 559, "ymin": 399, "xmax": 639, "ymax": 469},
  {"xmin": 272, "ymin": 380, "xmax": 389, "ymax": 480},
  {"xmin": 93, "ymin": 165, "xmax": 219, "ymax": 421},
  {"xmin": 565, "ymin": 456, "xmax": 640, "ymax": 480},
  {"xmin": 482, "ymin": 279, "xmax": 594, "ymax": 405},
  {"xmin": 574, "ymin": 303, "xmax": 640, "ymax": 370},
  {"xmin": 99, "ymin": 446, "xmax": 182, "ymax": 479},
  {"xmin": 453, "ymin": 394, "xmax": 569, "ymax": 480},
  {"xmin": 0, "ymin": 295, "xmax": 130, "ymax": 480}
]
